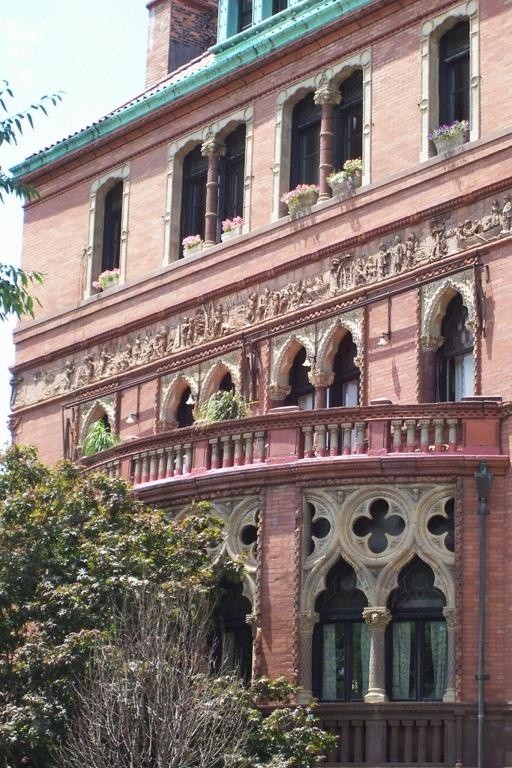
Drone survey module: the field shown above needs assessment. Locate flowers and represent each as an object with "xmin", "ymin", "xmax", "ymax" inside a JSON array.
[
  {"xmin": 280, "ymin": 183, "xmax": 319, "ymax": 225},
  {"xmin": 426, "ymin": 120, "xmax": 471, "ymax": 142},
  {"xmin": 327, "ymin": 171, "xmax": 353, "ymax": 197},
  {"xmin": 221, "ymin": 216, "xmax": 244, "ymax": 234},
  {"xmin": 343, "ymin": 157, "xmax": 362, "ymax": 178},
  {"xmin": 182, "ymin": 234, "xmax": 203, "ymax": 252},
  {"xmin": 91, "ymin": 267, "xmax": 120, "ymax": 290}
]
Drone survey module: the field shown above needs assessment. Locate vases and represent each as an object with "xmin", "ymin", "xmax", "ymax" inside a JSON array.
[
  {"xmin": 350, "ymin": 175, "xmax": 362, "ymax": 188},
  {"xmin": 434, "ymin": 135, "xmax": 465, "ymax": 155},
  {"xmin": 182, "ymin": 245, "xmax": 201, "ymax": 258},
  {"xmin": 221, "ymin": 229, "xmax": 239, "ymax": 243},
  {"xmin": 102, "ymin": 281, "xmax": 118, "ymax": 291},
  {"xmin": 331, "ymin": 182, "xmax": 347, "ymax": 197}
]
